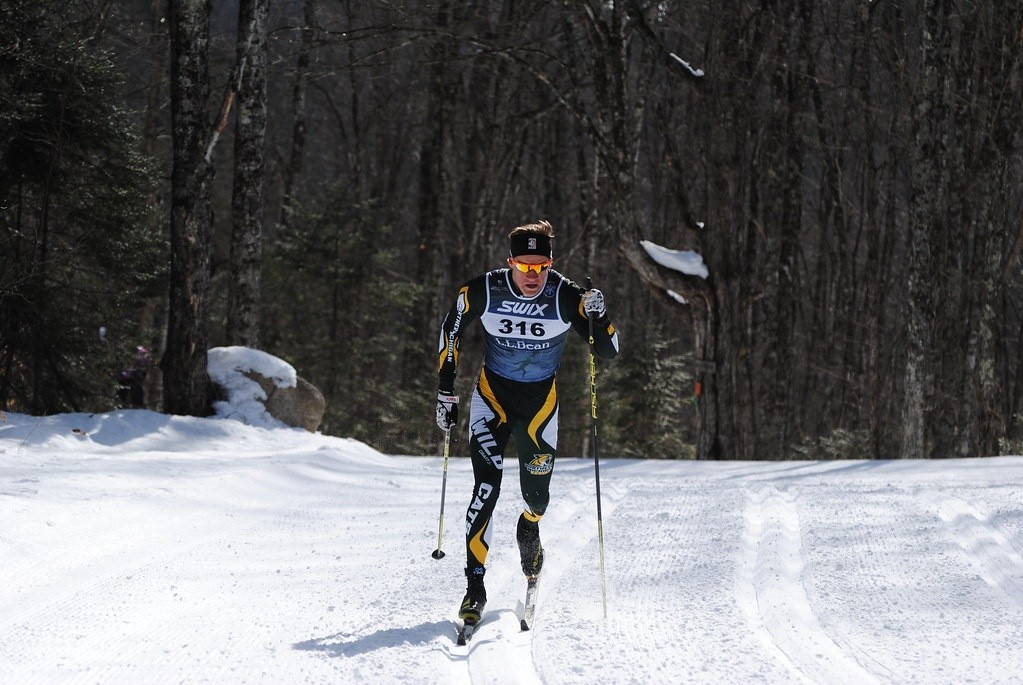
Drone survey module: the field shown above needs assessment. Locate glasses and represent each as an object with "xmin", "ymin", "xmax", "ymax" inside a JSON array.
[{"xmin": 510, "ymin": 258, "xmax": 553, "ymax": 275}]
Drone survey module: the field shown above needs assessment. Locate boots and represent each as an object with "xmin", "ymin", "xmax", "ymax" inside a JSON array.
[
  {"xmin": 458, "ymin": 568, "xmax": 488, "ymax": 622},
  {"xmin": 516, "ymin": 513, "xmax": 544, "ymax": 576}
]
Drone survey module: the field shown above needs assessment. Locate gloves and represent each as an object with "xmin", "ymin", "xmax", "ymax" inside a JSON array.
[
  {"xmin": 580, "ymin": 287, "xmax": 606, "ymax": 320},
  {"xmin": 436, "ymin": 394, "xmax": 460, "ymax": 432}
]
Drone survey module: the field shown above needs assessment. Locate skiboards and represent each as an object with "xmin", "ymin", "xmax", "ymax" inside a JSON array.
[{"xmin": 456, "ymin": 584, "xmax": 537, "ymax": 647}]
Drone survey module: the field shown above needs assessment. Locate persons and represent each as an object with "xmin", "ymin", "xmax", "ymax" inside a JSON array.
[{"xmin": 436, "ymin": 220, "xmax": 619, "ymax": 623}]
